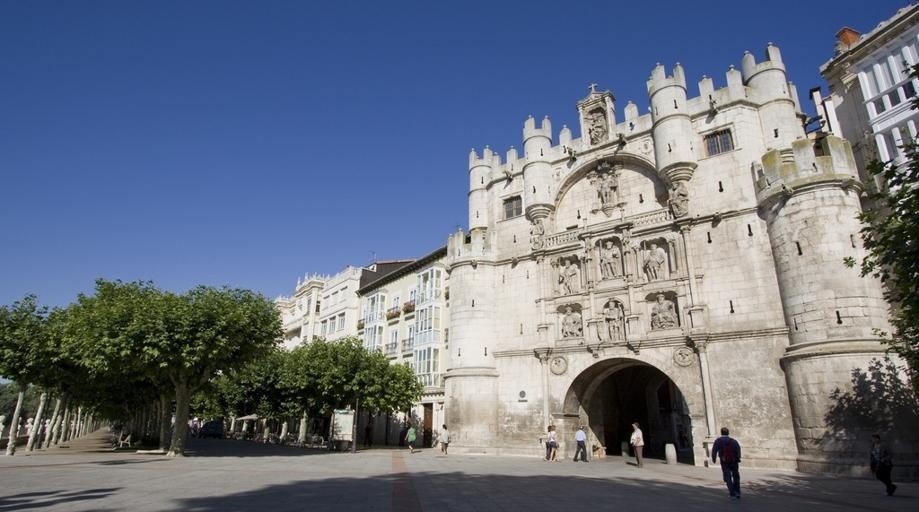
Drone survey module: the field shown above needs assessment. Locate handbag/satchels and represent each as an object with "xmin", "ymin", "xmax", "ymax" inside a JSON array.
[{"xmin": 722, "ymin": 445, "xmax": 735, "ymax": 463}]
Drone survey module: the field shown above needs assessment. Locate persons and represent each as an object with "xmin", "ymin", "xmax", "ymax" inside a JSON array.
[
  {"xmin": 629, "ymin": 421, "xmax": 645, "ymax": 468},
  {"xmin": 363, "ymin": 418, "xmax": 373, "ymax": 447},
  {"xmin": 648, "ymin": 292, "xmax": 680, "ymax": 332},
  {"xmin": 560, "ymin": 306, "xmax": 585, "ymax": 340},
  {"xmin": 546, "ymin": 425, "xmax": 560, "ymax": 462},
  {"xmin": 710, "ymin": 427, "xmax": 742, "ymax": 499},
  {"xmin": 600, "ymin": 301, "xmax": 625, "ymax": 343},
  {"xmin": 557, "ymin": 257, "xmax": 582, "ymax": 295},
  {"xmin": 529, "ymin": 220, "xmax": 545, "ymax": 263},
  {"xmin": 597, "ymin": 171, "xmax": 618, "ymax": 204},
  {"xmin": 433, "ymin": 424, "xmax": 452, "ymax": 454},
  {"xmin": 668, "ymin": 181, "xmax": 690, "ymax": 218},
  {"xmin": 598, "ymin": 243, "xmax": 624, "ymax": 284},
  {"xmin": 869, "ymin": 431, "xmax": 898, "ymax": 496},
  {"xmin": 544, "ymin": 425, "xmax": 551, "ymax": 462},
  {"xmin": 584, "ymin": 113, "xmax": 607, "ymax": 144},
  {"xmin": 640, "ymin": 242, "xmax": 671, "ymax": 278},
  {"xmin": 572, "ymin": 426, "xmax": 592, "ymax": 462},
  {"xmin": 404, "ymin": 421, "xmax": 418, "ymax": 454},
  {"xmin": 107, "ymin": 414, "xmax": 344, "ymax": 450}
]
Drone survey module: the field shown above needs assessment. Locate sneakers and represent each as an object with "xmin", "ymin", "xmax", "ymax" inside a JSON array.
[{"xmin": 728, "ymin": 492, "xmax": 740, "ymax": 500}]
